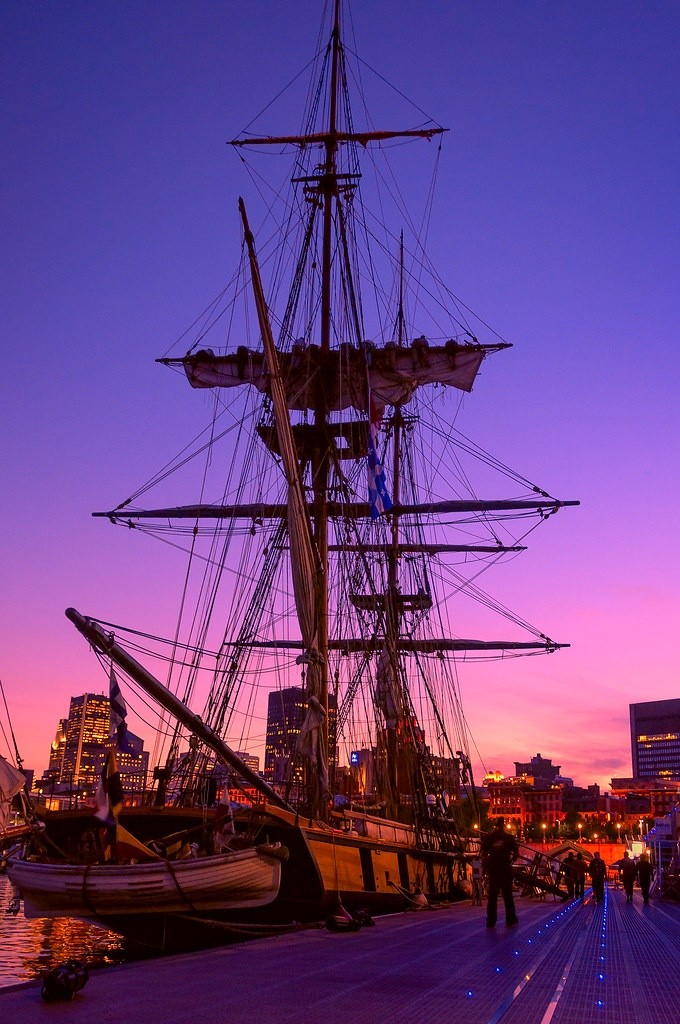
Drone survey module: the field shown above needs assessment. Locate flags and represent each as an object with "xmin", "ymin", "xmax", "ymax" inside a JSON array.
[
  {"xmin": 366, "ymin": 422, "xmax": 393, "ymax": 522},
  {"xmin": 109, "ymin": 666, "xmax": 128, "ymax": 749}
]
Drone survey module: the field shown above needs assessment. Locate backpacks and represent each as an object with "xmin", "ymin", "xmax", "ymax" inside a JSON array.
[{"xmin": 479, "ymin": 839, "xmax": 507, "ymax": 877}]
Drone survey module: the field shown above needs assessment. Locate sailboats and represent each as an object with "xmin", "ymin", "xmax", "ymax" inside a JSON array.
[{"xmin": 0, "ymin": 20, "xmax": 586, "ymax": 944}]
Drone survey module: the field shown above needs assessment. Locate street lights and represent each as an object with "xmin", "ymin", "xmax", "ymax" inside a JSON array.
[
  {"xmin": 577, "ymin": 823, "xmax": 584, "ymax": 845},
  {"xmin": 542, "ymin": 824, "xmax": 547, "ymax": 844},
  {"xmin": 617, "ymin": 823, "xmax": 622, "ymax": 844}
]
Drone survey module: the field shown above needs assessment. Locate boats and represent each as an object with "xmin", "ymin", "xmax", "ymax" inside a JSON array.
[{"xmin": 5, "ymin": 843, "xmax": 292, "ymax": 920}]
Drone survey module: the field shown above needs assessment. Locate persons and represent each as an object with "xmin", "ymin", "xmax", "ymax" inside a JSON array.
[
  {"xmin": 620, "ymin": 852, "xmax": 636, "ymax": 903},
  {"xmin": 637, "ymin": 854, "xmax": 654, "ymax": 905},
  {"xmin": 483, "ymin": 817, "xmax": 519, "ymax": 928},
  {"xmin": 561, "ymin": 850, "xmax": 576, "ymax": 898},
  {"xmin": 589, "ymin": 850, "xmax": 608, "ymax": 903},
  {"xmin": 575, "ymin": 852, "xmax": 590, "ymax": 900}
]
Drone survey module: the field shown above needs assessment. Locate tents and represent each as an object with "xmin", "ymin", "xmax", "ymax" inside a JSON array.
[{"xmin": 610, "ymin": 856, "xmax": 637, "ymax": 889}]
